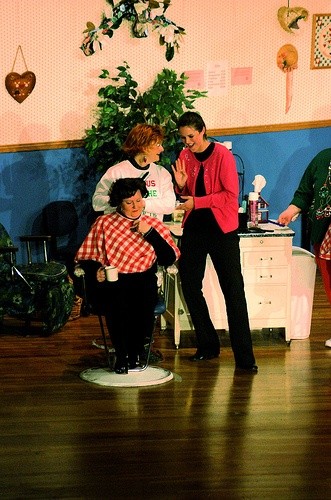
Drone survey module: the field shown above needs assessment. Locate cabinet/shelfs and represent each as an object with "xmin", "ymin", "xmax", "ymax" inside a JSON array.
[{"xmin": 160, "ymin": 219, "xmax": 295, "ymax": 349}]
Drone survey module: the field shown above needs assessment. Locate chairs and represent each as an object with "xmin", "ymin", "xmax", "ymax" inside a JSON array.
[
  {"xmin": 79, "ymin": 295, "xmax": 174, "ymax": 387},
  {"xmin": 45, "ymin": 201, "xmax": 90, "ymax": 316},
  {"xmin": 0, "ymin": 223, "xmax": 75, "ymax": 336}
]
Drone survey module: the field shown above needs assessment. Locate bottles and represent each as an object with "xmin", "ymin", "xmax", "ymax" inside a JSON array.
[{"xmin": 248, "ymin": 192, "xmax": 258, "ymax": 227}]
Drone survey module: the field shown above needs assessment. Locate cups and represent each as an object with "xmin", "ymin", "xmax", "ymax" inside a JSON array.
[{"xmin": 105, "ymin": 265, "xmax": 118, "ymax": 282}]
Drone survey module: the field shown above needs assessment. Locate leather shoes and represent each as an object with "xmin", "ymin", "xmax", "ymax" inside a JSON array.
[
  {"xmin": 114, "ymin": 352, "xmax": 144, "ymax": 374},
  {"xmin": 190, "ymin": 350, "xmax": 217, "ymax": 361},
  {"xmin": 234, "ymin": 365, "xmax": 257, "ymax": 375}
]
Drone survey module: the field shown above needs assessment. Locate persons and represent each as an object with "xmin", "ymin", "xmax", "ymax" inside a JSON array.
[
  {"xmin": 170, "ymin": 112, "xmax": 258, "ymax": 373},
  {"xmin": 278, "ymin": 148, "xmax": 331, "ymax": 348},
  {"xmin": 77, "ymin": 177, "xmax": 181, "ymax": 375},
  {"xmin": 91, "ymin": 124, "xmax": 177, "ymax": 361}
]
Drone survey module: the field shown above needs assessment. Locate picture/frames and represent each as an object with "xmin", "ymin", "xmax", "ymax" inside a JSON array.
[{"xmin": 310, "ymin": 14, "xmax": 331, "ymax": 69}]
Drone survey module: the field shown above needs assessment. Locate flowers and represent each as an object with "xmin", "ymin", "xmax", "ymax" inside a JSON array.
[{"xmin": 79, "ymin": 0, "xmax": 187, "ymax": 62}]
